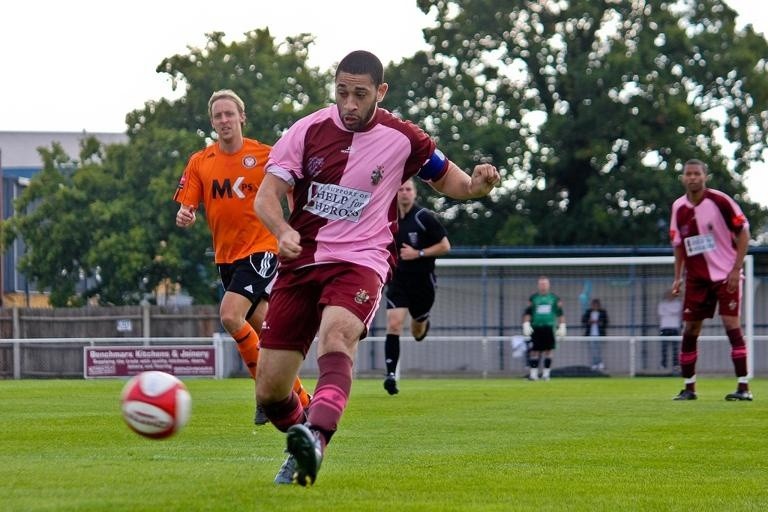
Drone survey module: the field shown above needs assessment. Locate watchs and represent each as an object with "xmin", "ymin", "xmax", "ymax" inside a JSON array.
[{"xmin": 418, "ymin": 248, "xmax": 426, "ymax": 257}]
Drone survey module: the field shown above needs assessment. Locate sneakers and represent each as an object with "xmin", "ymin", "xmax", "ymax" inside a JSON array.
[
  {"xmin": 725, "ymin": 390, "xmax": 753, "ymax": 400},
  {"xmin": 672, "ymin": 389, "xmax": 697, "ymax": 400},
  {"xmin": 254, "ymin": 394, "xmax": 322, "ymax": 486},
  {"xmin": 384, "ymin": 374, "xmax": 399, "ymax": 395}
]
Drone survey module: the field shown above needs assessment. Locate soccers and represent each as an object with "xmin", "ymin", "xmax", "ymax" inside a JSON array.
[{"xmin": 122, "ymin": 371, "xmax": 190, "ymax": 440}]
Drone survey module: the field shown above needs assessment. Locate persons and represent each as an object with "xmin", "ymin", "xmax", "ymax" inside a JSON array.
[
  {"xmin": 669, "ymin": 159, "xmax": 753, "ymax": 401},
  {"xmin": 254, "ymin": 52, "xmax": 502, "ymax": 486},
  {"xmin": 382, "ymin": 178, "xmax": 451, "ymax": 395},
  {"xmin": 521, "ymin": 276, "xmax": 567, "ymax": 380},
  {"xmin": 657, "ymin": 290, "xmax": 684, "ymax": 369},
  {"xmin": 172, "ymin": 89, "xmax": 314, "ymax": 425},
  {"xmin": 582, "ymin": 298, "xmax": 608, "ymax": 370}
]
